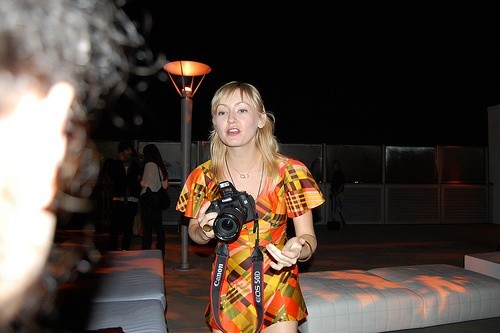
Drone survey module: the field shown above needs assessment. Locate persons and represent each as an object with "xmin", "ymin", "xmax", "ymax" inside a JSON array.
[
  {"xmin": 103, "ymin": 142, "xmax": 168, "ymax": 257},
  {"xmin": 328, "ymin": 160, "xmax": 345, "ymax": 225},
  {"xmin": 0, "ymin": 0, "xmax": 138, "ymax": 311},
  {"xmin": 176, "ymin": 81, "xmax": 324, "ymax": 333}
]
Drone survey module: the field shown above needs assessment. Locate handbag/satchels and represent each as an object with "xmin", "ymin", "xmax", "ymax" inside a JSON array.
[{"xmin": 139, "ymin": 186, "xmax": 172, "ymax": 213}]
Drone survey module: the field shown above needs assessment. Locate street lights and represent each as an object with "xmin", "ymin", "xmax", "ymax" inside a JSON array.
[{"xmin": 163, "ymin": 60, "xmax": 212, "ymax": 272}]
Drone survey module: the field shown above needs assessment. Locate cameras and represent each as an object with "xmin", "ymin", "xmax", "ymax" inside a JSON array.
[{"xmin": 205, "ymin": 181, "xmax": 257, "ymax": 243}]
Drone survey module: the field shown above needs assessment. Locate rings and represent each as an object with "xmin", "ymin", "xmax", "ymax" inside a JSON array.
[{"xmin": 203, "ymin": 224, "xmax": 211, "ymax": 232}]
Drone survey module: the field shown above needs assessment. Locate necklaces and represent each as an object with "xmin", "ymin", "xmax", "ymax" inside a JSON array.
[
  {"xmin": 232, "ymin": 159, "xmax": 257, "ymax": 179},
  {"xmin": 225, "ymin": 151, "xmax": 264, "ymax": 197}
]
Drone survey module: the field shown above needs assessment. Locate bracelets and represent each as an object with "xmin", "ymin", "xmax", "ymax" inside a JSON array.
[{"xmin": 298, "ymin": 241, "xmax": 312, "ymax": 262}]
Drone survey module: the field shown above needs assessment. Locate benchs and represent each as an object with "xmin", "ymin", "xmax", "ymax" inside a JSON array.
[
  {"xmin": 297, "ymin": 262, "xmax": 500, "ymax": 333},
  {"xmin": 84, "ymin": 249, "xmax": 169, "ymax": 333}
]
